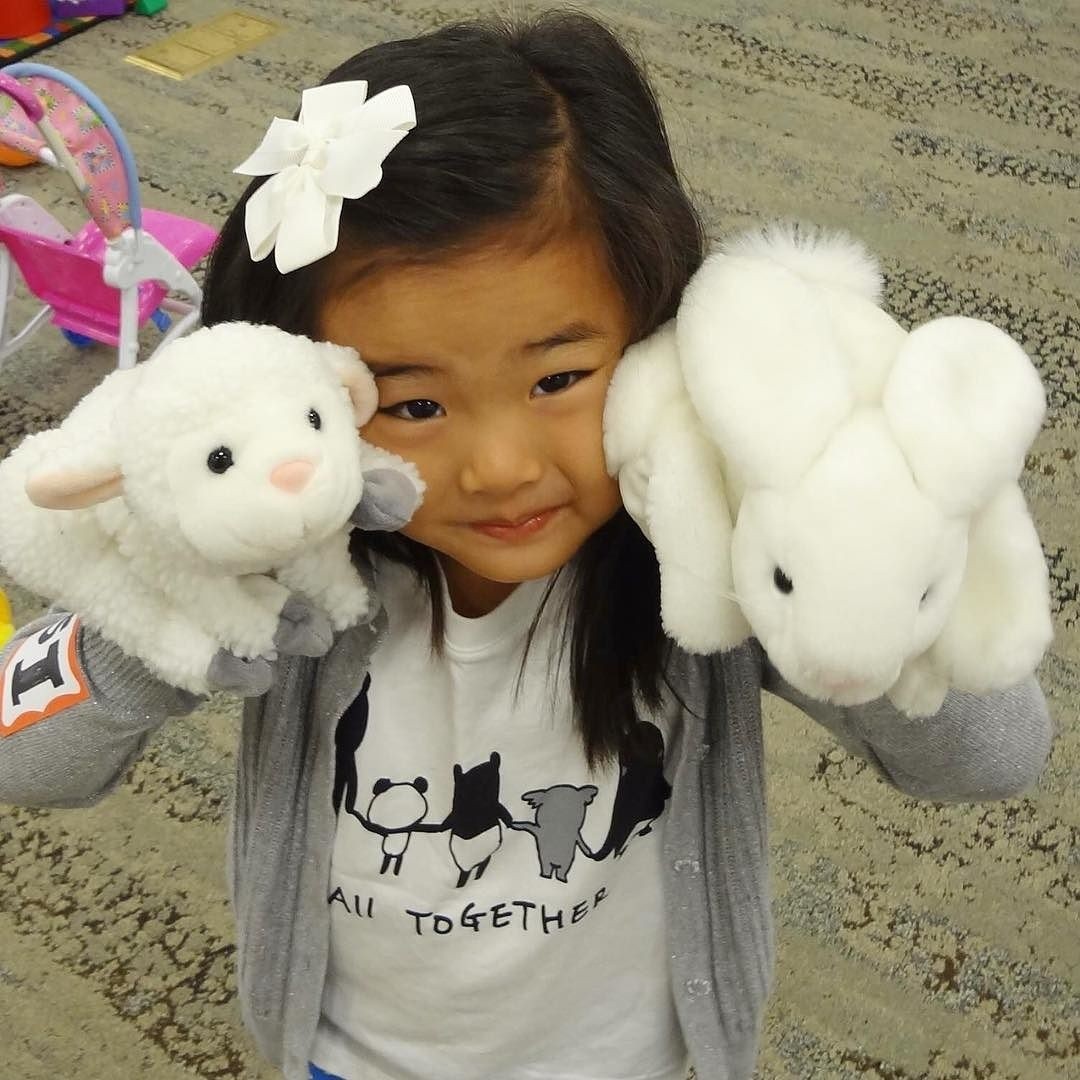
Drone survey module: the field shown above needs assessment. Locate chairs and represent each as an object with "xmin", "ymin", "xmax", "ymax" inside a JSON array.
[{"xmin": 0, "ymin": 74, "xmax": 220, "ymax": 378}]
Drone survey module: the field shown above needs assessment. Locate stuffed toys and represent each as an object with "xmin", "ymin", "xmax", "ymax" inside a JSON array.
[
  {"xmin": 602, "ymin": 222, "xmax": 1057, "ymax": 719},
  {"xmin": 0, "ymin": 321, "xmax": 428, "ymax": 699}
]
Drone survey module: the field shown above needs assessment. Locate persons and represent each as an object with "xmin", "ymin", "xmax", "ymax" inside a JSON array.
[{"xmin": 0, "ymin": 10, "xmax": 1055, "ymax": 1079}]
